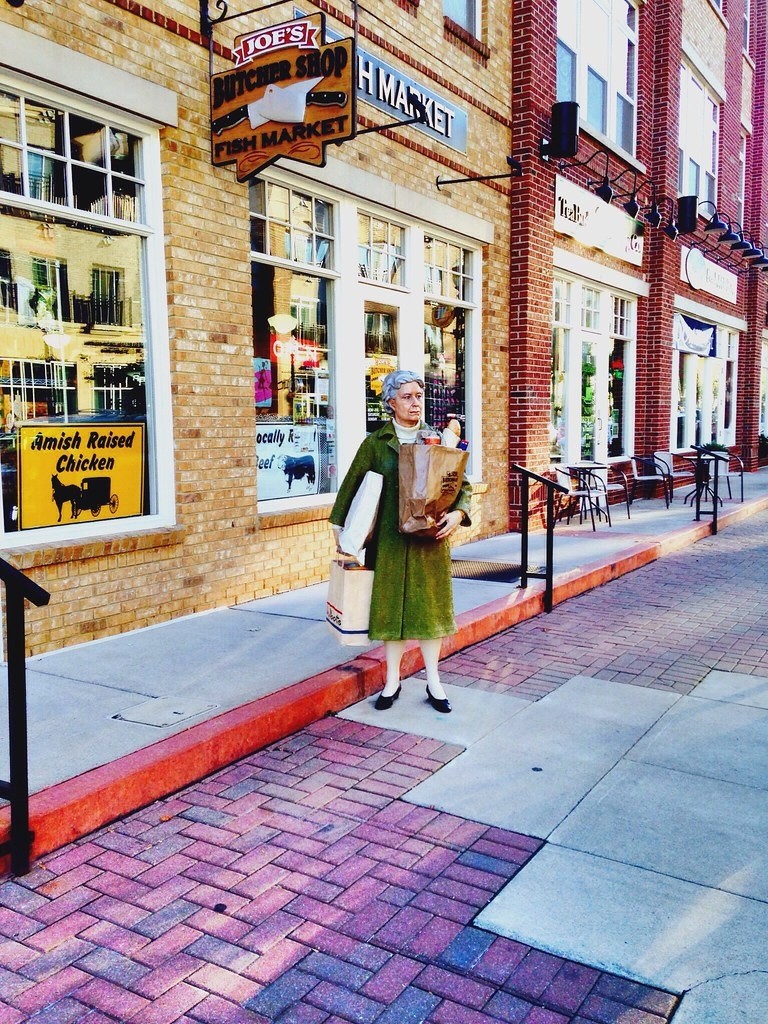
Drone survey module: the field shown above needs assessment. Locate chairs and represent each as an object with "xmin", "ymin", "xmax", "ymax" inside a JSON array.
[
  {"xmin": 626, "ymin": 453, "xmax": 674, "ymax": 509},
  {"xmin": 551, "ymin": 465, "xmax": 613, "ymax": 533},
  {"xmin": 701, "ymin": 450, "xmax": 744, "ymax": 503},
  {"xmin": 584, "ymin": 460, "xmax": 631, "ymax": 528},
  {"xmin": 653, "ymin": 450, "xmax": 699, "ymax": 505}
]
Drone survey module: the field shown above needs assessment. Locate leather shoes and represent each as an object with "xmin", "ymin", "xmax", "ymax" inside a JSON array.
[
  {"xmin": 425, "ymin": 685, "xmax": 452, "ymax": 713},
  {"xmin": 375, "ymin": 682, "xmax": 401, "ymax": 710}
]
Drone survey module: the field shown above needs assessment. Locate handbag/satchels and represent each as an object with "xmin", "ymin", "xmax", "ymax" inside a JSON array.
[
  {"xmin": 337, "ymin": 470, "xmax": 383, "ymax": 557},
  {"xmin": 325, "ymin": 549, "xmax": 374, "ymax": 647},
  {"xmin": 399, "ymin": 431, "xmax": 470, "ymax": 540}
]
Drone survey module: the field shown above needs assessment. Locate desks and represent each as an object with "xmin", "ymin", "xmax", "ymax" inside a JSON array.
[
  {"xmin": 681, "ymin": 455, "xmax": 723, "ymax": 507},
  {"xmin": 566, "ymin": 464, "xmax": 609, "ymax": 525}
]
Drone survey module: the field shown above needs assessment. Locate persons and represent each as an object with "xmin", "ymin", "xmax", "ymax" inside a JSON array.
[{"xmin": 329, "ymin": 370, "xmax": 472, "ymax": 713}]
